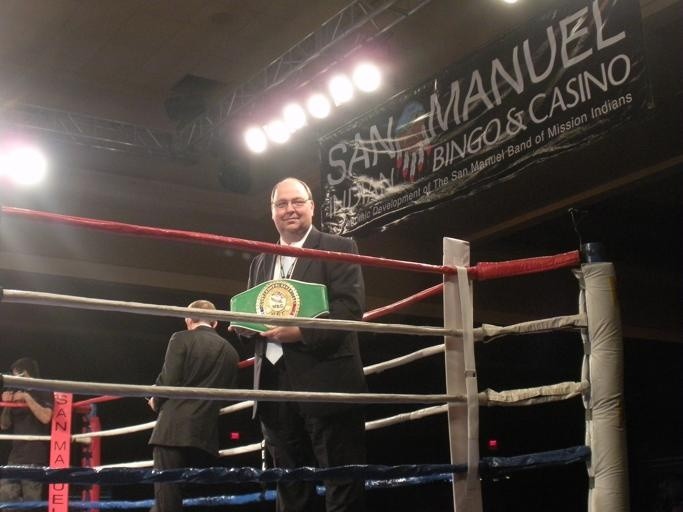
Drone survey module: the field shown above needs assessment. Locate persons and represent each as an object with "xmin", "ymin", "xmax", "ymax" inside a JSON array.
[
  {"xmin": 0, "ymin": 357, "xmax": 55, "ymax": 512},
  {"xmin": 148, "ymin": 300, "xmax": 240, "ymax": 511},
  {"xmin": 227, "ymin": 176, "xmax": 370, "ymax": 511}
]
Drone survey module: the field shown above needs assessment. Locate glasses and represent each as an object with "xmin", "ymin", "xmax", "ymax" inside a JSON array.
[{"xmin": 272, "ymin": 198, "xmax": 310, "ymax": 208}]
